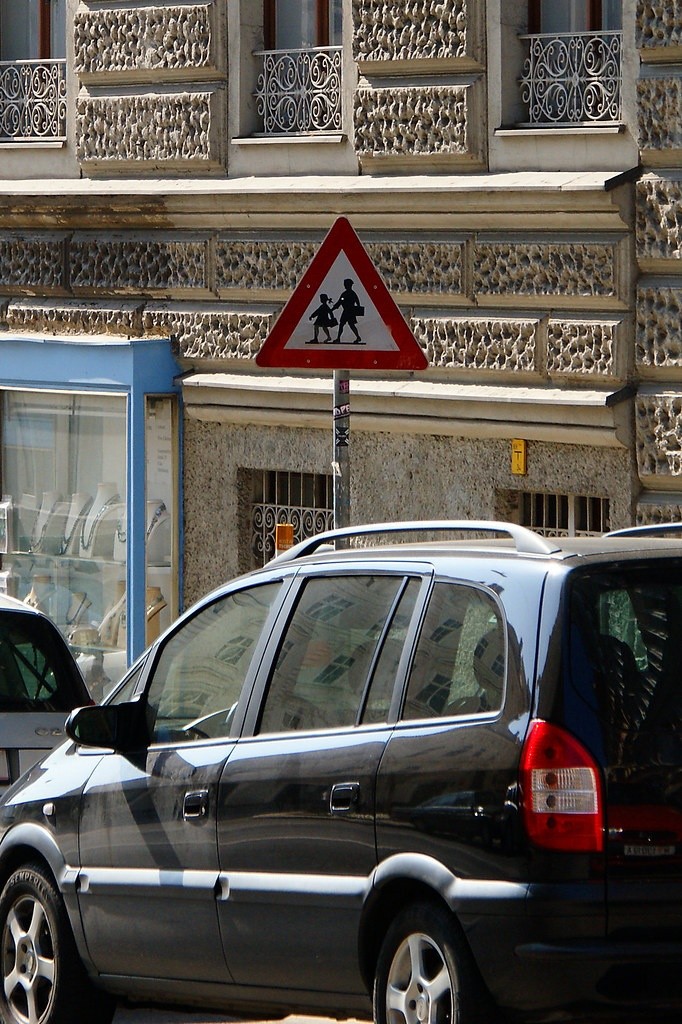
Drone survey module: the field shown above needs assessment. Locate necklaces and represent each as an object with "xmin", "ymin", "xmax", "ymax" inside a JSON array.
[
  {"xmin": 65, "ymin": 592, "xmax": 87, "ymax": 625},
  {"xmin": 81, "ymin": 494, "xmax": 120, "ymax": 549},
  {"xmin": 61, "ymin": 498, "xmax": 93, "ymax": 554},
  {"xmin": 31, "ymin": 496, "xmax": 62, "ymax": 547},
  {"xmin": 89, "ymin": 671, "xmax": 108, "ymax": 693},
  {"xmin": 121, "ymin": 595, "xmax": 164, "ymax": 620},
  {"xmin": 26, "ymin": 584, "xmax": 55, "ymax": 607},
  {"xmin": 117, "ymin": 503, "xmax": 166, "ymax": 542},
  {"xmin": 106, "ymin": 599, "xmax": 126, "ymax": 639}
]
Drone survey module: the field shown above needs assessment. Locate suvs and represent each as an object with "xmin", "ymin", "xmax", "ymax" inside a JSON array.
[{"xmin": 0, "ymin": 520, "xmax": 682, "ymax": 1024}]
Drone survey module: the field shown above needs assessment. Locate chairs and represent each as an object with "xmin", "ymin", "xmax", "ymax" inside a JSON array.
[
  {"xmin": 443, "ymin": 629, "xmax": 504, "ymax": 716},
  {"xmin": 335, "ymin": 637, "xmax": 432, "ymax": 726}
]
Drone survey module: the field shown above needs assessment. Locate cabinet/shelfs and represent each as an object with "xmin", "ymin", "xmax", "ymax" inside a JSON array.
[{"xmin": 0, "ymin": 551, "xmax": 171, "ymax": 698}]
